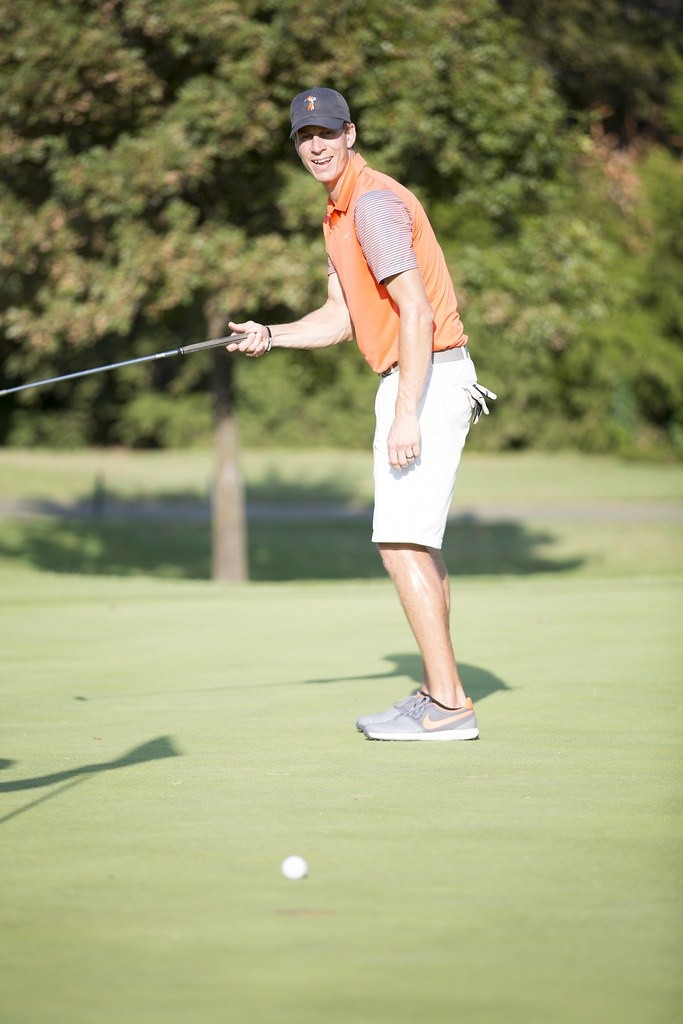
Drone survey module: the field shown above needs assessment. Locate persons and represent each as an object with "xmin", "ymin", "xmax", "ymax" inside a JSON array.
[{"xmin": 227, "ymin": 87, "xmax": 498, "ymax": 739}]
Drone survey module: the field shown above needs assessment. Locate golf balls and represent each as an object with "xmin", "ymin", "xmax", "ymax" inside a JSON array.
[{"xmin": 281, "ymin": 855, "xmax": 306, "ymax": 880}]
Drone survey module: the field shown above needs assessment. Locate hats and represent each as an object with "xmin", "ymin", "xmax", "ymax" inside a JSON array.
[{"xmin": 288, "ymin": 85, "xmax": 352, "ymax": 138}]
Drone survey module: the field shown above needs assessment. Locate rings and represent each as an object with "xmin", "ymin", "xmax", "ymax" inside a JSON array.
[{"xmin": 406, "ymin": 455, "xmax": 414, "ymax": 459}]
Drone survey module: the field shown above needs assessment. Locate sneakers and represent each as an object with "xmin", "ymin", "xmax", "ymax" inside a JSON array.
[
  {"xmin": 356, "ymin": 694, "xmax": 429, "ymax": 730},
  {"xmin": 363, "ymin": 700, "xmax": 480, "ymax": 742}
]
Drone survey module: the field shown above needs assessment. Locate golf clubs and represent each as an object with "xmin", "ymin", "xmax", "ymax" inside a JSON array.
[{"xmin": 0, "ymin": 332, "xmax": 253, "ymax": 395}]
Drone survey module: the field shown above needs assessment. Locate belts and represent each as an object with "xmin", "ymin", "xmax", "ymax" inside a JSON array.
[{"xmin": 377, "ymin": 343, "xmax": 469, "ymax": 378}]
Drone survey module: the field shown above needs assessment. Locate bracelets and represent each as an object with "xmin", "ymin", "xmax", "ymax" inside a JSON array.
[{"xmin": 265, "ymin": 327, "xmax": 272, "ymax": 354}]
